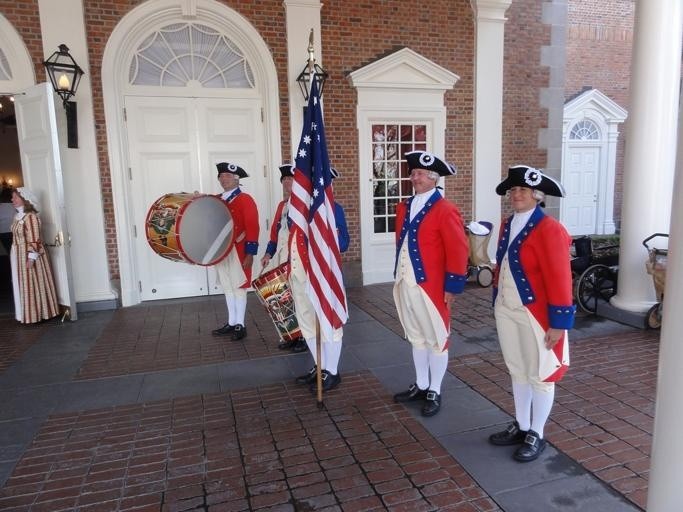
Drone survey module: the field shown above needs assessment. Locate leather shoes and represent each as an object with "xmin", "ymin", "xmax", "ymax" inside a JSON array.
[
  {"xmin": 393, "ymin": 382, "xmax": 430, "ymax": 402},
  {"xmin": 512, "ymin": 430, "xmax": 547, "ymax": 463},
  {"xmin": 420, "ymin": 390, "xmax": 441, "ymax": 416},
  {"xmin": 210, "ymin": 321, "xmax": 234, "ymax": 336},
  {"xmin": 488, "ymin": 420, "xmax": 531, "ymax": 447},
  {"xmin": 277, "ymin": 336, "xmax": 308, "ymax": 352},
  {"xmin": 230, "ymin": 323, "xmax": 248, "ymax": 341},
  {"xmin": 295, "ymin": 365, "xmax": 342, "ymax": 394}
]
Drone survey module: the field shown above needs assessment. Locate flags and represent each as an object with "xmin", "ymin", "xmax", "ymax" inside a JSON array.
[{"xmin": 285, "ymin": 72, "xmax": 349, "ymax": 330}]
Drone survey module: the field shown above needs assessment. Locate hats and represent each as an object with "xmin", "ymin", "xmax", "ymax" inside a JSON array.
[
  {"xmin": 278, "ymin": 164, "xmax": 300, "ymax": 185},
  {"xmin": 495, "ymin": 165, "xmax": 568, "ymax": 199},
  {"xmin": 402, "ymin": 150, "xmax": 458, "ymax": 177},
  {"xmin": 216, "ymin": 162, "xmax": 249, "ymax": 179}
]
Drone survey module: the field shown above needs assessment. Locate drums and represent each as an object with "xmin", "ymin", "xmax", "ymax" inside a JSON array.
[
  {"xmin": 251, "ymin": 262, "xmax": 308, "ymax": 353},
  {"xmin": 145, "ymin": 193, "xmax": 237, "ymax": 266}
]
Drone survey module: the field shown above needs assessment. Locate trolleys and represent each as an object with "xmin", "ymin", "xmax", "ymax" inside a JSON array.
[
  {"xmin": 461, "ymin": 219, "xmax": 497, "ymax": 287},
  {"xmin": 561, "ymin": 232, "xmax": 617, "ymax": 315}
]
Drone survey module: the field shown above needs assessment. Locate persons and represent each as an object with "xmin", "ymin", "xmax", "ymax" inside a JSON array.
[
  {"xmin": 391, "ymin": 150, "xmax": 471, "ymax": 418},
  {"xmin": 211, "ymin": 161, "xmax": 260, "ymax": 341},
  {"xmin": 8, "ymin": 187, "xmax": 61, "ymax": 325},
  {"xmin": 260, "ymin": 164, "xmax": 308, "ymax": 352},
  {"xmin": 295, "ymin": 168, "xmax": 350, "ymax": 393},
  {"xmin": 488, "ymin": 164, "xmax": 578, "ymax": 462}
]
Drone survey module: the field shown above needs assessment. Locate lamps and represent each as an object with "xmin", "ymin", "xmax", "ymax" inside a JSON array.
[
  {"xmin": 43, "ymin": 44, "xmax": 85, "ymax": 109},
  {"xmin": 297, "ymin": 57, "xmax": 328, "ymax": 100}
]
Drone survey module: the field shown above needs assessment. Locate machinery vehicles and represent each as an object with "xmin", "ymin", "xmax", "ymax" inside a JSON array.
[{"xmin": 641, "ymin": 231, "xmax": 669, "ymax": 330}]
